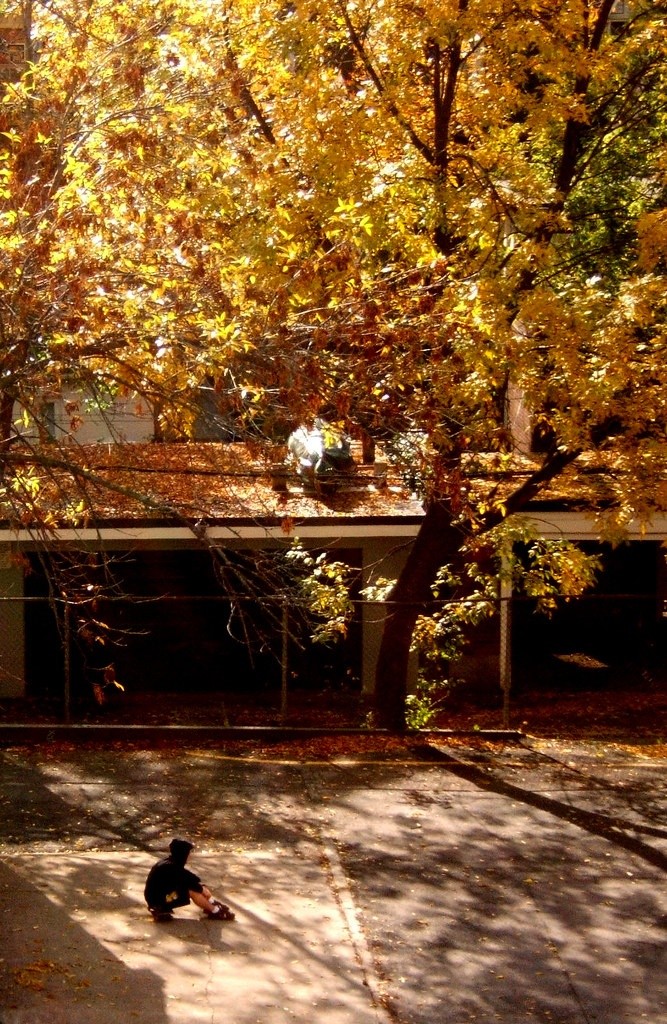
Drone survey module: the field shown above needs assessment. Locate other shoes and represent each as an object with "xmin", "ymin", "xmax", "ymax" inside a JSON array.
[
  {"xmin": 203, "ymin": 900, "xmax": 229, "ymax": 913},
  {"xmin": 207, "ymin": 905, "xmax": 235, "ymax": 921}
]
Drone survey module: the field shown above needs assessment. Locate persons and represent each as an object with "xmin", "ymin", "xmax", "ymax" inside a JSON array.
[{"xmin": 145, "ymin": 839, "xmax": 236, "ymax": 919}]
